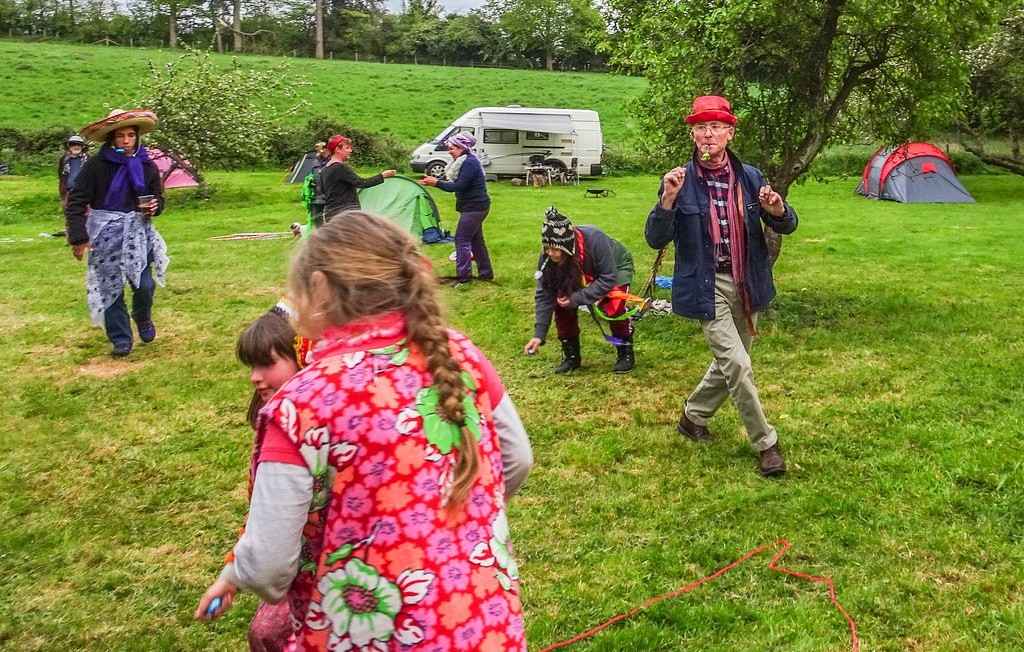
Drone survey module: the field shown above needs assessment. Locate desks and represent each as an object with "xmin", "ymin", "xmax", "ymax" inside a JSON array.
[{"xmin": 525, "ymin": 166, "xmax": 552, "ymax": 186}]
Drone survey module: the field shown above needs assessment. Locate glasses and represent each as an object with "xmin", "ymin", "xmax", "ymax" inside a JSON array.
[{"xmin": 691, "ymin": 125, "xmax": 730, "ymax": 134}]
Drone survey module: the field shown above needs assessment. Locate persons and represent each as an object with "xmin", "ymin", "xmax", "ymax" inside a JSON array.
[
  {"xmin": 58, "ymin": 135, "xmax": 94, "ymax": 246},
  {"xmin": 320, "ymin": 135, "xmax": 397, "ymax": 223},
  {"xmin": 643, "ymin": 95, "xmax": 799, "ymax": 478},
  {"xmin": 232, "ymin": 209, "xmax": 535, "ymax": 652},
  {"xmin": 192, "ymin": 295, "xmax": 321, "ymax": 652},
  {"xmin": 524, "ymin": 205, "xmax": 636, "ymax": 374},
  {"xmin": 418, "ymin": 130, "xmax": 495, "ymax": 284},
  {"xmin": 63, "ymin": 108, "xmax": 171, "ymax": 357}
]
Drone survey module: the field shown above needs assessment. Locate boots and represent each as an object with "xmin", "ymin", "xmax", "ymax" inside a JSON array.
[
  {"xmin": 613, "ymin": 326, "xmax": 635, "ymax": 374},
  {"xmin": 555, "ymin": 330, "xmax": 582, "ymax": 374}
]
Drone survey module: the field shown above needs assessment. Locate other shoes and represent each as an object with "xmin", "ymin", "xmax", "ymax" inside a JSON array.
[
  {"xmin": 478, "ymin": 277, "xmax": 494, "ymax": 281},
  {"xmin": 450, "ymin": 281, "xmax": 472, "ymax": 288}
]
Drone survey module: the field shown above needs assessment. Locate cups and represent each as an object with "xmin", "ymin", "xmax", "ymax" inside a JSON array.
[{"xmin": 138, "ymin": 195, "xmax": 154, "ymax": 214}]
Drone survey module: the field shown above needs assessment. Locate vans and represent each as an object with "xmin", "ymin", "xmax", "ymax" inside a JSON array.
[{"xmin": 410, "ymin": 104, "xmax": 604, "ymax": 180}]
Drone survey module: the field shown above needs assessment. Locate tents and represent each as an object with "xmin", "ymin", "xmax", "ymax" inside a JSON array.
[
  {"xmin": 280, "ymin": 145, "xmax": 331, "ymax": 183},
  {"xmin": 852, "ymin": 141, "xmax": 977, "ymax": 204},
  {"xmin": 356, "ymin": 175, "xmax": 445, "ymax": 243},
  {"xmin": 141, "ymin": 140, "xmax": 206, "ymax": 189}
]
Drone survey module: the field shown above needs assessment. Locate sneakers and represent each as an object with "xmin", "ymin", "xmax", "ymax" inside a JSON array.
[
  {"xmin": 131, "ymin": 310, "xmax": 156, "ymax": 343},
  {"xmin": 110, "ymin": 345, "xmax": 132, "ymax": 356}
]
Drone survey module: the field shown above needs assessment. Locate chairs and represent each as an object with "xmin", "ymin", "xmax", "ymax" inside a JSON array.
[{"xmin": 559, "ymin": 158, "xmax": 580, "ymax": 187}]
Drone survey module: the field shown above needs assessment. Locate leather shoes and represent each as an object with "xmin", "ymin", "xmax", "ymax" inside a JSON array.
[
  {"xmin": 761, "ymin": 439, "xmax": 786, "ymax": 476},
  {"xmin": 678, "ymin": 411, "xmax": 714, "ymax": 444}
]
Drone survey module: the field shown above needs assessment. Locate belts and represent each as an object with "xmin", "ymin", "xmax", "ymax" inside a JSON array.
[{"xmin": 715, "ymin": 265, "xmax": 732, "ymax": 273}]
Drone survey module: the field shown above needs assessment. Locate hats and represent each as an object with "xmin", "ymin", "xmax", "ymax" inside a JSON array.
[
  {"xmin": 534, "ymin": 206, "xmax": 585, "ymax": 280},
  {"xmin": 79, "ymin": 109, "xmax": 159, "ymax": 142},
  {"xmin": 448, "ymin": 131, "xmax": 476, "ymax": 151},
  {"xmin": 685, "ymin": 95, "xmax": 737, "ymax": 126},
  {"xmin": 65, "ymin": 136, "xmax": 89, "ymax": 153}
]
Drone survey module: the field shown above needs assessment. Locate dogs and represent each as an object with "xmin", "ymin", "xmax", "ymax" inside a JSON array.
[{"xmin": 290, "ymin": 221, "xmax": 307, "ymax": 237}]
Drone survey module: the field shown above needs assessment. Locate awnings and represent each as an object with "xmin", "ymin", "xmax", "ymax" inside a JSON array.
[{"xmin": 481, "ymin": 112, "xmax": 572, "ymax": 135}]
